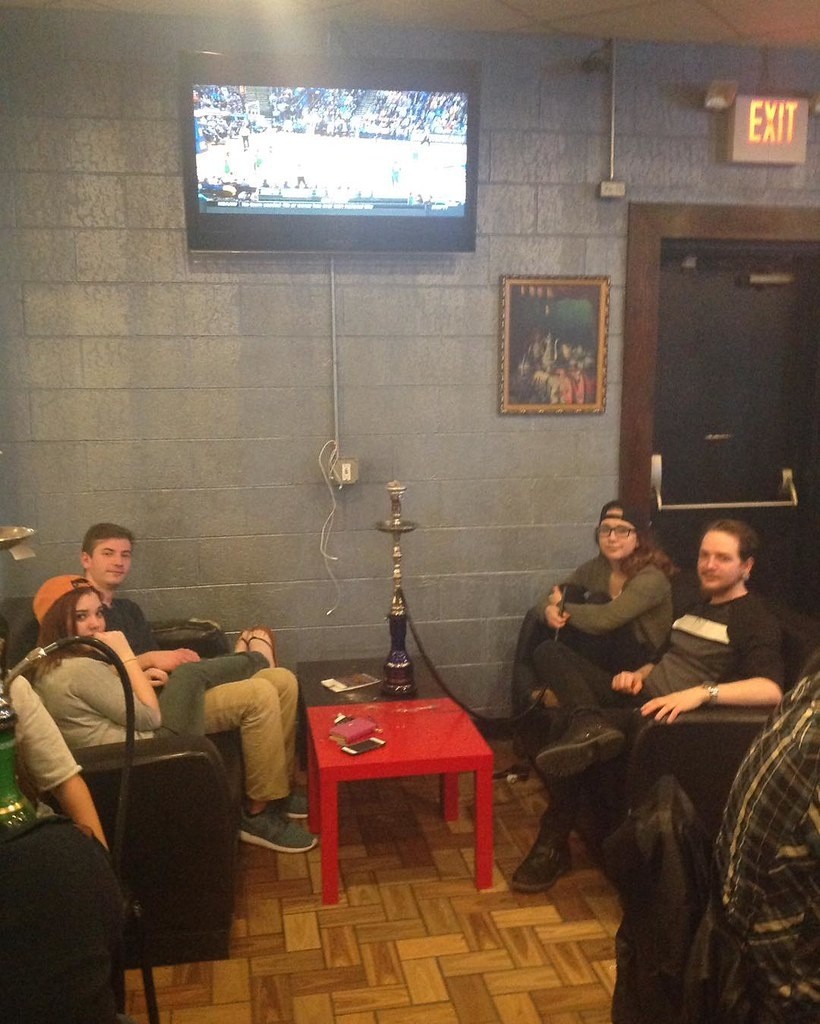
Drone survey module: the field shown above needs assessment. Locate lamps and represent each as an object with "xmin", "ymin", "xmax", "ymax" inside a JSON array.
[{"xmin": 703, "ymin": 77, "xmax": 820, "ymax": 164}]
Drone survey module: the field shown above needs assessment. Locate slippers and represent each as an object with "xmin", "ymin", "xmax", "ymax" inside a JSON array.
[
  {"xmin": 235, "ymin": 628, "xmax": 251, "ymax": 652},
  {"xmin": 248, "ymin": 626, "xmax": 278, "ymax": 669}
]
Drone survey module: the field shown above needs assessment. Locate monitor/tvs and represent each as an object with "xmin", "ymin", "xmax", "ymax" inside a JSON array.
[{"xmin": 177, "ymin": 49, "xmax": 481, "ymax": 254}]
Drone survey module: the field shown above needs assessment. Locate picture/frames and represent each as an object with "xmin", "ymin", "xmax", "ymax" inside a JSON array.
[{"xmin": 499, "ymin": 274, "xmax": 611, "ymax": 414}]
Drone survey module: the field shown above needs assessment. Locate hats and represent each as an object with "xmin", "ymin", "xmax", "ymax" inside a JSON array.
[
  {"xmin": 32, "ymin": 574, "xmax": 94, "ymax": 625},
  {"xmin": 600, "ymin": 499, "xmax": 648, "ymax": 532}
]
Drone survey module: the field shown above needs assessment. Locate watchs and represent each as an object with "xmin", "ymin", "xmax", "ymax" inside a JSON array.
[{"xmin": 703, "ymin": 681, "xmax": 718, "ymax": 709}]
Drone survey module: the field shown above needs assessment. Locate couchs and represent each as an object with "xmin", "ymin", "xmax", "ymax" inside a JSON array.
[
  {"xmin": 513, "ymin": 572, "xmax": 820, "ymax": 888},
  {"xmin": 0, "ymin": 596, "xmax": 239, "ymax": 963}
]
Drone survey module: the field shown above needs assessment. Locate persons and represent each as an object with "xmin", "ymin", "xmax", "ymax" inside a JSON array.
[
  {"xmin": 0, "ymin": 612, "xmax": 111, "ymax": 853},
  {"xmin": 193, "ymin": 84, "xmax": 468, "ymax": 212},
  {"xmin": 511, "ymin": 519, "xmax": 786, "ymax": 894},
  {"xmin": 527, "ymin": 498, "xmax": 681, "ymax": 709},
  {"xmin": 708, "ymin": 645, "xmax": 820, "ymax": 1024},
  {"xmin": 30, "ymin": 574, "xmax": 277, "ymax": 749},
  {"xmin": 81, "ymin": 522, "xmax": 319, "ymax": 854}
]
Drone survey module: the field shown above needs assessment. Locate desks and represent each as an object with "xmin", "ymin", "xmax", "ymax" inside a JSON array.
[{"xmin": 297, "ymin": 654, "xmax": 494, "ymax": 903}]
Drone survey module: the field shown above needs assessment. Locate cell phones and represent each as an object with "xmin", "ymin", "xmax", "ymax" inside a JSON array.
[{"xmin": 341, "ymin": 737, "xmax": 385, "ymax": 755}]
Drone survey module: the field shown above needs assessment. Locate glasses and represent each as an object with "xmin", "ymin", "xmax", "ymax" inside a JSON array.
[{"xmin": 595, "ymin": 525, "xmax": 636, "ymax": 538}]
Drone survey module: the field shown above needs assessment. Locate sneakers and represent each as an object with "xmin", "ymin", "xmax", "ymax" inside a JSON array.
[
  {"xmin": 511, "ymin": 833, "xmax": 572, "ymax": 890},
  {"xmin": 534, "ymin": 714, "xmax": 625, "ymax": 777},
  {"xmin": 276, "ymin": 792, "xmax": 309, "ymax": 819},
  {"xmin": 239, "ymin": 807, "xmax": 318, "ymax": 853}
]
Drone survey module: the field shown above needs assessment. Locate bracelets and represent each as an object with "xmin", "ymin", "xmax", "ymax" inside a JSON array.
[{"xmin": 123, "ymin": 658, "xmax": 138, "ymax": 664}]
